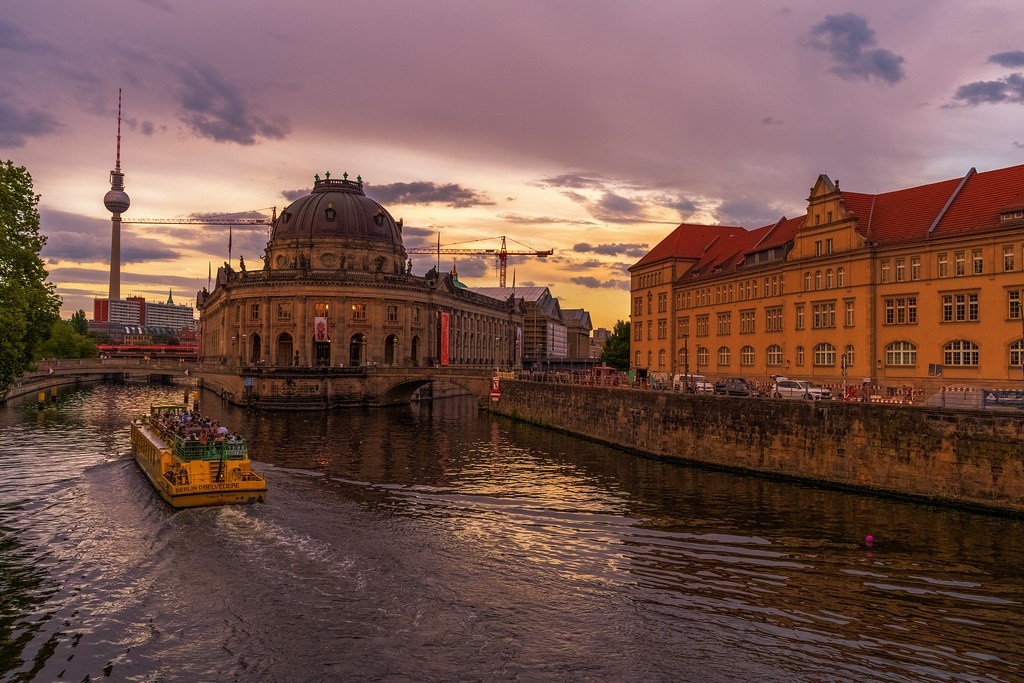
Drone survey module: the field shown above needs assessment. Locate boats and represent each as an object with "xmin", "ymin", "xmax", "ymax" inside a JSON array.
[{"xmin": 129, "ymin": 400, "xmax": 269, "ymax": 511}]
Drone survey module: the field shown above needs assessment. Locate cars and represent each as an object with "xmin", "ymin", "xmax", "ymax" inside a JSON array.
[
  {"xmin": 713, "ymin": 376, "xmax": 760, "ymax": 397},
  {"xmin": 769, "ymin": 376, "xmax": 833, "ymax": 399}
]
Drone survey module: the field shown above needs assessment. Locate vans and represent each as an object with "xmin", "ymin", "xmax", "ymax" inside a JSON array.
[{"xmin": 672, "ymin": 373, "xmax": 714, "ymax": 394}]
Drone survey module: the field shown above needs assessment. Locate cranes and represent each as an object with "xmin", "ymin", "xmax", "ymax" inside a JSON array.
[
  {"xmin": 404, "ymin": 234, "xmax": 553, "ymax": 287},
  {"xmin": 121, "ymin": 206, "xmax": 280, "ymax": 238}
]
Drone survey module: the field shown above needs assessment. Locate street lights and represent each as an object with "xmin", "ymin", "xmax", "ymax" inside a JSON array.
[
  {"xmin": 538, "ymin": 344, "xmax": 543, "ymax": 372},
  {"xmin": 515, "ymin": 341, "xmax": 520, "ymax": 371},
  {"xmin": 592, "ymin": 350, "xmax": 596, "ymax": 374},
  {"xmin": 494, "ymin": 337, "xmax": 500, "ymax": 371},
  {"xmin": 681, "ymin": 333, "xmax": 690, "ymax": 393}
]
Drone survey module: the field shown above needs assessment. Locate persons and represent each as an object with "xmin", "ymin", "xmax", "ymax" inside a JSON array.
[
  {"xmin": 756, "ymin": 382, "xmax": 763, "ymax": 395},
  {"xmin": 406, "ymin": 259, "xmax": 412, "ymax": 272},
  {"xmin": 377, "ymin": 255, "xmax": 383, "ymax": 270},
  {"xmin": 299, "ymin": 252, "xmax": 305, "ymax": 267},
  {"xmin": 340, "ymin": 253, "xmax": 346, "ymax": 268},
  {"xmin": 688, "ymin": 378, "xmax": 697, "ymax": 394},
  {"xmin": 849, "ymin": 385, "xmax": 858, "ymax": 401},
  {"xmin": 556, "ymin": 371, "xmax": 619, "ymax": 386},
  {"xmin": 367, "ymin": 360, "xmax": 379, "ymax": 367},
  {"xmin": 861, "ymin": 382, "xmax": 868, "ymax": 402},
  {"xmin": 154, "ymin": 409, "xmax": 246, "ymax": 458},
  {"xmin": 655, "ymin": 374, "xmax": 664, "ymax": 389},
  {"xmin": 240, "ymin": 256, "xmax": 244, "ymax": 269}
]
